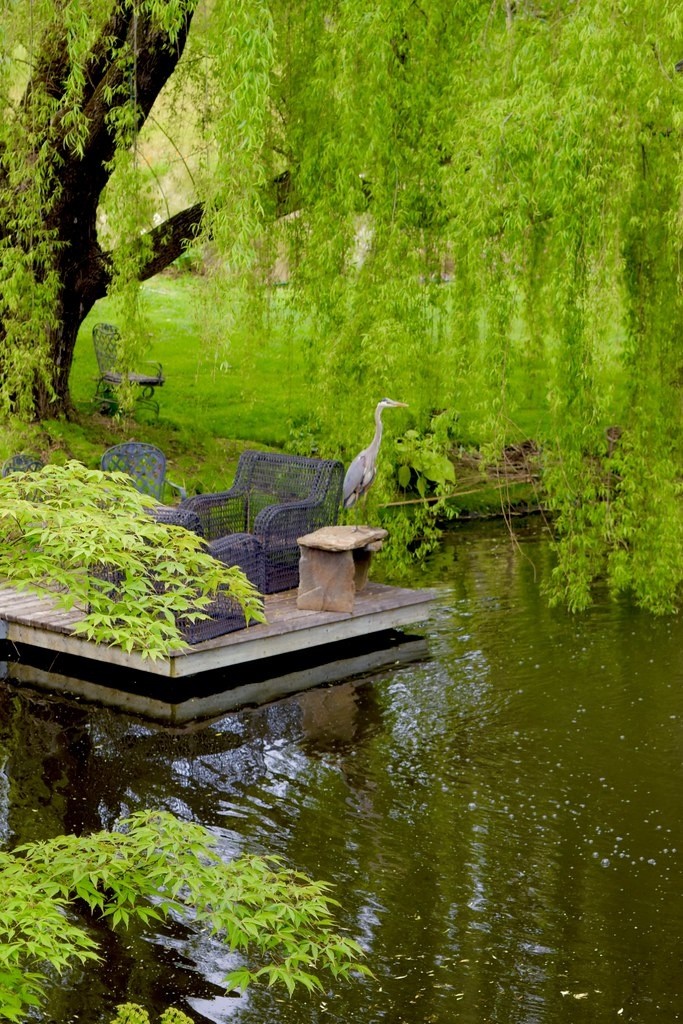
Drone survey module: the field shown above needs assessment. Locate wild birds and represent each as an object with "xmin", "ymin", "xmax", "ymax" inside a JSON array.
[{"xmin": 343, "ymin": 398, "xmax": 409, "ymax": 534}]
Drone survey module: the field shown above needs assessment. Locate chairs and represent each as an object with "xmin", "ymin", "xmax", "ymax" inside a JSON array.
[
  {"xmin": 90, "ymin": 323, "xmax": 165, "ymax": 420},
  {"xmin": 2, "ymin": 454, "xmax": 48, "ymax": 503},
  {"xmin": 178, "ymin": 449, "xmax": 346, "ymax": 594},
  {"xmin": 102, "ymin": 441, "xmax": 188, "ymax": 505},
  {"xmin": 87, "ymin": 505, "xmax": 267, "ymax": 645}
]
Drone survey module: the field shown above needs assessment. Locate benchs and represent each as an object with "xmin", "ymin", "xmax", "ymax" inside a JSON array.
[{"xmin": 296, "ymin": 525, "xmax": 388, "ymax": 614}]
